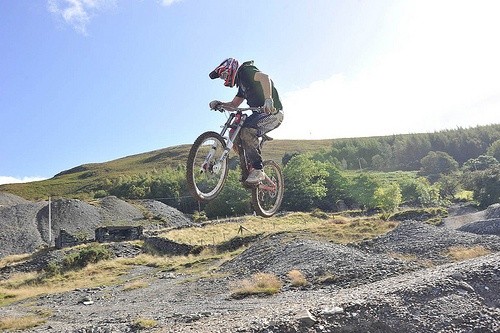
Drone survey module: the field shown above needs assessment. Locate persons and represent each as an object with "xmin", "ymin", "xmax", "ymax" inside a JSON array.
[{"xmin": 210, "ymin": 57, "xmax": 284, "ymax": 184}]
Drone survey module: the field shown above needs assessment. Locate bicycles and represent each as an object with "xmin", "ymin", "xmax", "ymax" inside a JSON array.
[{"xmin": 185, "ymin": 102, "xmax": 285, "ymax": 218}]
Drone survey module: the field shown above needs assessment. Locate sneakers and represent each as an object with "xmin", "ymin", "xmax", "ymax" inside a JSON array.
[{"xmin": 245, "ymin": 169, "xmax": 265, "ymax": 186}]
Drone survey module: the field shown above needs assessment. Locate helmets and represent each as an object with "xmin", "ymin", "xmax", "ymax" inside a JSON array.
[{"xmin": 209, "ymin": 58, "xmax": 238, "ymax": 87}]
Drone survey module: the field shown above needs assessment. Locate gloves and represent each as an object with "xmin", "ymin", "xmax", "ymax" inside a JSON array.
[
  {"xmin": 209, "ymin": 100, "xmax": 223, "ymax": 112},
  {"xmin": 263, "ymin": 99, "xmax": 275, "ymax": 115}
]
